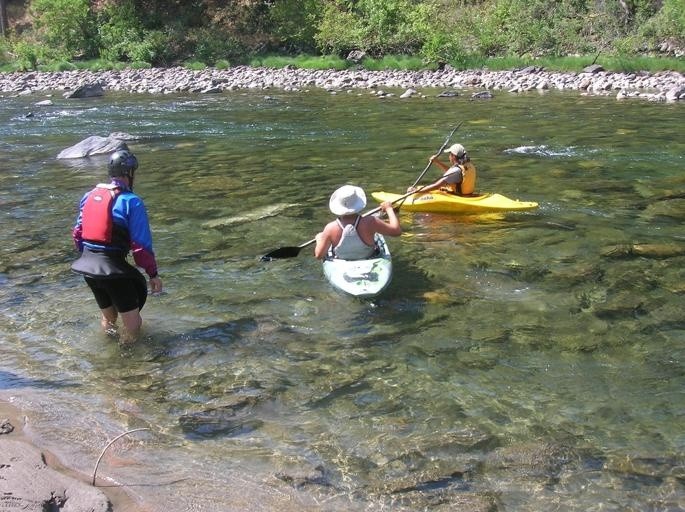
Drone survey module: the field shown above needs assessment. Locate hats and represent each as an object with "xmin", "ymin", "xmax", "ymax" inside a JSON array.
[
  {"xmin": 443, "ymin": 144, "xmax": 466, "ymax": 158},
  {"xmin": 329, "ymin": 185, "xmax": 367, "ymax": 217}
]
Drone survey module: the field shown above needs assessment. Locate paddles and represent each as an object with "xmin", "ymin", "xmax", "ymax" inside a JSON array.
[
  {"xmin": 381, "ymin": 120, "xmax": 464, "ymax": 218},
  {"xmin": 260, "ymin": 185, "xmax": 428, "ymax": 261}
]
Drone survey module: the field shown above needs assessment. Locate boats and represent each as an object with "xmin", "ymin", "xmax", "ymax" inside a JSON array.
[
  {"xmin": 370, "ymin": 189, "xmax": 541, "ymax": 215},
  {"xmin": 320, "ymin": 231, "xmax": 395, "ymax": 299}
]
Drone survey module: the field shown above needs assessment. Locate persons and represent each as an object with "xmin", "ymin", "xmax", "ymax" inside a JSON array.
[
  {"xmin": 69, "ymin": 148, "xmax": 162, "ymax": 341},
  {"xmin": 311, "ymin": 186, "xmax": 403, "ymax": 260},
  {"xmin": 408, "ymin": 143, "xmax": 478, "ymax": 197}
]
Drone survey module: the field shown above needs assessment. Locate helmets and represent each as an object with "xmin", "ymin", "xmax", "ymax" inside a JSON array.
[{"xmin": 109, "ymin": 150, "xmax": 138, "ymax": 178}]
